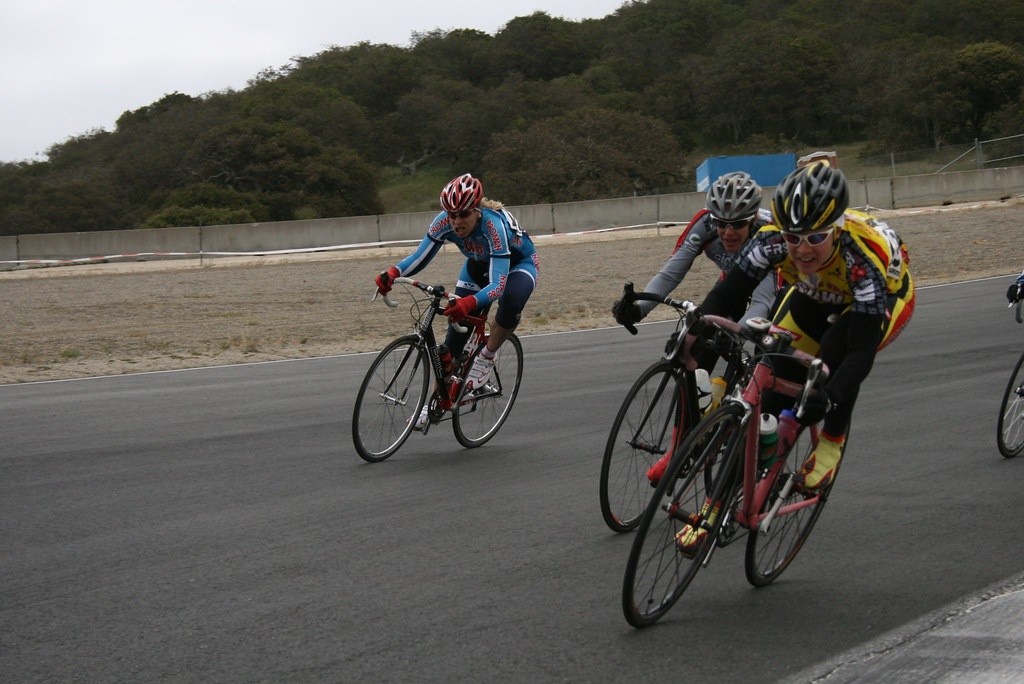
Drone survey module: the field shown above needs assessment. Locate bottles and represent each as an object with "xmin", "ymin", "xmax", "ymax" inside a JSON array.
[
  {"xmin": 695, "ymin": 369, "xmax": 712, "ymax": 414},
  {"xmin": 710, "ymin": 376, "xmax": 727, "ymax": 411},
  {"xmin": 777, "ymin": 408, "xmax": 801, "ymax": 457},
  {"xmin": 437, "ymin": 344, "xmax": 453, "ymax": 379},
  {"xmin": 760, "ymin": 413, "xmax": 778, "ymax": 472}
]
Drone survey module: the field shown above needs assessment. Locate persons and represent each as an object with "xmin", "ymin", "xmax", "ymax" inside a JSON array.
[
  {"xmin": 1007, "ymin": 270, "xmax": 1024, "ymax": 303},
  {"xmin": 374, "ymin": 173, "xmax": 541, "ymax": 430},
  {"xmin": 611, "ymin": 170, "xmax": 786, "ymax": 484},
  {"xmin": 675, "ymin": 159, "xmax": 916, "ymax": 557}
]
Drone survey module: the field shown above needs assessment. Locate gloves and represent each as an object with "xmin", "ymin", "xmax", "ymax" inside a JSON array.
[
  {"xmin": 613, "ymin": 299, "xmax": 642, "ymax": 327},
  {"xmin": 794, "ymin": 395, "xmax": 828, "ymax": 425},
  {"xmin": 664, "ymin": 331, "xmax": 706, "ymax": 359},
  {"xmin": 1007, "ymin": 283, "xmax": 1024, "ymax": 305},
  {"xmin": 445, "ymin": 295, "xmax": 477, "ymax": 322},
  {"xmin": 374, "ymin": 266, "xmax": 401, "ymax": 296}
]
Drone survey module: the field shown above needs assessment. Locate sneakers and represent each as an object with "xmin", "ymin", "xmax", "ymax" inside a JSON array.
[
  {"xmin": 466, "ymin": 353, "xmax": 498, "ymax": 389},
  {"xmin": 793, "ymin": 432, "xmax": 848, "ymax": 490},
  {"xmin": 647, "ymin": 428, "xmax": 677, "ymax": 478},
  {"xmin": 674, "ymin": 498, "xmax": 722, "ymax": 551},
  {"xmin": 406, "ymin": 405, "xmax": 431, "ymax": 432}
]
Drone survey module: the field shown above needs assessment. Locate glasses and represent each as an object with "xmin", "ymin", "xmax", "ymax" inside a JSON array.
[
  {"xmin": 448, "ymin": 210, "xmax": 474, "ymax": 220},
  {"xmin": 780, "ymin": 228, "xmax": 832, "ymax": 246},
  {"xmin": 710, "ymin": 212, "xmax": 755, "ymax": 230}
]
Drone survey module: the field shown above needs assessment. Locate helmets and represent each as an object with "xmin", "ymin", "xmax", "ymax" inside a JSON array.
[
  {"xmin": 770, "ymin": 160, "xmax": 849, "ymax": 234},
  {"xmin": 440, "ymin": 173, "xmax": 484, "ymax": 214},
  {"xmin": 706, "ymin": 171, "xmax": 763, "ymax": 220}
]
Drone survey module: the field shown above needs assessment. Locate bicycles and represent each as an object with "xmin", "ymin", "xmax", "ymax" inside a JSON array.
[
  {"xmin": 351, "ymin": 269, "xmax": 525, "ymax": 463},
  {"xmin": 996, "ymin": 281, "xmax": 1024, "ymax": 458},
  {"xmin": 599, "ymin": 279, "xmax": 733, "ymax": 534},
  {"xmin": 619, "ymin": 309, "xmax": 845, "ymax": 628}
]
